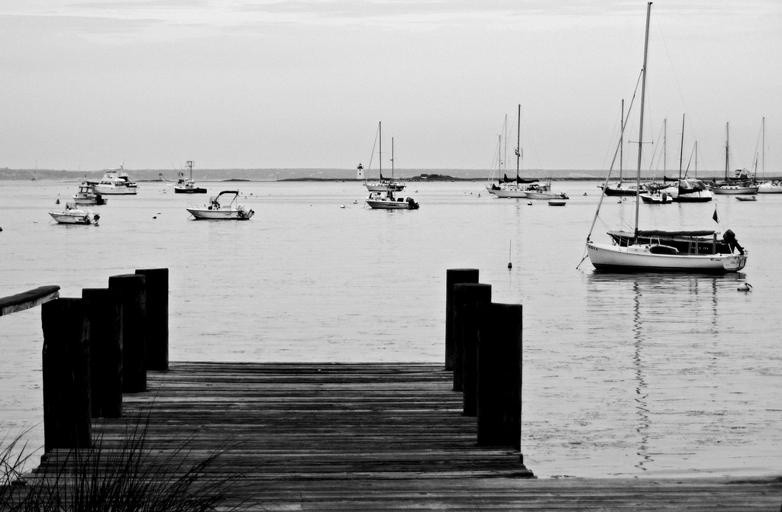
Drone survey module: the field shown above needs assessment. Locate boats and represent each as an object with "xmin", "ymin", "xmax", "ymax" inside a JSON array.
[
  {"xmin": 81, "ymin": 167, "xmax": 138, "ymax": 196},
  {"xmin": 186, "ymin": 191, "xmax": 255, "ymax": 220},
  {"xmin": 525, "ymin": 190, "xmax": 569, "ymax": 200},
  {"xmin": 548, "ymin": 202, "xmax": 566, "ymax": 206},
  {"xmin": 73, "ymin": 185, "xmax": 107, "ymax": 204},
  {"xmin": 50, "ymin": 202, "xmax": 100, "ymax": 227},
  {"xmin": 174, "ymin": 160, "xmax": 208, "ymax": 194}
]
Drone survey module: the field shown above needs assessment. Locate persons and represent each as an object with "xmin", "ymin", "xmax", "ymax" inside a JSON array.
[{"xmin": 369, "ymin": 192, "xmax": 381, "ymax": 200}]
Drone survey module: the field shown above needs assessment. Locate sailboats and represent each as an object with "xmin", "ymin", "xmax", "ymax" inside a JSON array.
[
  {"xmin": 599, "ymin": 97, "xmax": 782, "ymax": 205},
  {"xmin": 364, "ymin": 121, "xmax": 406, "ymax": 193},
  {"xmin": 367, "ymin": 136, "xmax": 420, "ymax": 209},
  {"xmin": 585, "ymin": 0, "xmax": 747, "ymax": 273},
  {"xmin": 486, "ymin": 104, "xmax": 552, "ymax": 199}
]
